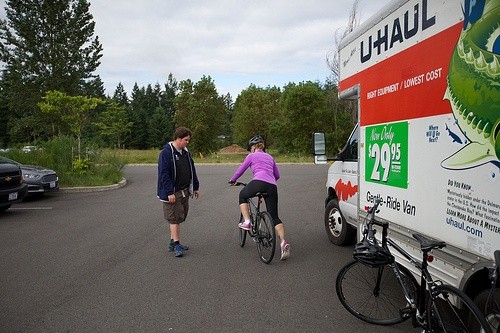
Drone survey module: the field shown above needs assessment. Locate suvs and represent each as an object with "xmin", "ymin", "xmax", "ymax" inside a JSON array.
[{"xmin": 0, "ymin": 156, "xmax": 29, "ymax": 211}]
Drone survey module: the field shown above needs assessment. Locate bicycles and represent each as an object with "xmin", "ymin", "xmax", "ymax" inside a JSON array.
[
  {"xmin": 227, "ymin": 180, "xmax": 276, "ymax": 264},
  {"xmin": 337, "ymin": 197, "xmax": 493, "ymax": 333}
]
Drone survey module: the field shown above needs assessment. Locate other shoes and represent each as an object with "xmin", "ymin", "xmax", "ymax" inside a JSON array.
[
  {"xmin": 174, "ymin": 244, "xmax": 183, "ymax": 257},
  {"xmin": 168, "ymin": 244, "xmax": 189, "ymax": 252},
  {"xmin": 238, "ymin": 222, "xmax": 251, "ymax": 230},
  {"xmin": 280, "ymin": 240, "xmax": 291, "ymax": 261}
]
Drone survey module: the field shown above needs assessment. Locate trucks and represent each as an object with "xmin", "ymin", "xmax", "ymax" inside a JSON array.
[{"xmin": 311, "ymin": 1, "xmax": 500, "ymax": 333}]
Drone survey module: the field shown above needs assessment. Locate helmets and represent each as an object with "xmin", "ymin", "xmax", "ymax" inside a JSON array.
[
  {"xmin": 248, "ymin": 135, "xmax": 264, "ymax": 145},
  {"xmin": 352, "ymin": 241, "xmax": 392, "ymax": 269}
]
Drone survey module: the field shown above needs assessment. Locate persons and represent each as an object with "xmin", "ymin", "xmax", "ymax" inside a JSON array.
[
  {"xmin": 157, "ymin": 128, "xmax": 200, "ymax": 257},
  {"xmin": 230, "ymin": 135, "xmax": 290, "ymax": 261}
]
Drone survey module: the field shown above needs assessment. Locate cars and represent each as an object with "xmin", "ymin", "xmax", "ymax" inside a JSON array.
[{"xmin": 19, "ymin": 165, "xmax": 59, "ymax": 194}]
